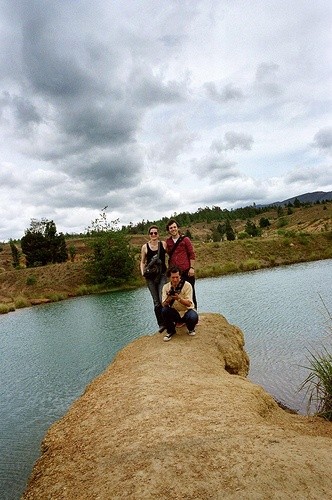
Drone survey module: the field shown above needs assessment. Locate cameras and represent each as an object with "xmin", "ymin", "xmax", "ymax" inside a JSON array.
[{"xmin": 169, "ymin": 287, "xmax": 177, "ymax": 296}]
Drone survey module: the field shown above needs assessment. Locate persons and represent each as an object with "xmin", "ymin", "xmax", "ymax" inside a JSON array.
[
  {"xmin": 140, "ymin": 225, "xmax": 167, "ymax": 327},
  {"xmin": 163, "ymin": 268, "xmax": 199, "ymax": 341},
  {"xmin": 165, "ymin": 220, "xmax": 197, "ymax": 327}
]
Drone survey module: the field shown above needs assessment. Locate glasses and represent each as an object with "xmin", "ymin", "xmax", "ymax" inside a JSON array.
[{"xmin": 149, "ymin": 232, "xmax": 157, "ymax": 235}]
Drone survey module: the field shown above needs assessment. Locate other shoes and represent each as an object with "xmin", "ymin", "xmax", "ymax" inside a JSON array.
[
  {"xmin": 188, "ymin": 328, "xmax": 196, "ymax": 336},
  {"xmin": 158, "ymin": 325, "xmax": 165, "ymax": 334},
  {"xmin": 163, "ymin": 330, "xmax": 176, "ymax": 342}
]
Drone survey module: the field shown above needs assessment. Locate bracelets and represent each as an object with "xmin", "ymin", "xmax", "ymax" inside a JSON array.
[{"xmin": 190, "ymin": 267, "xmax": 194, "ymax": 269}]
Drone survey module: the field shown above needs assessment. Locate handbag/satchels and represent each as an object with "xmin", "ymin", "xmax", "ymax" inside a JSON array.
[{"xmin": 142, "ymin": 242, "xmax": 163, "ymax": 285}]
